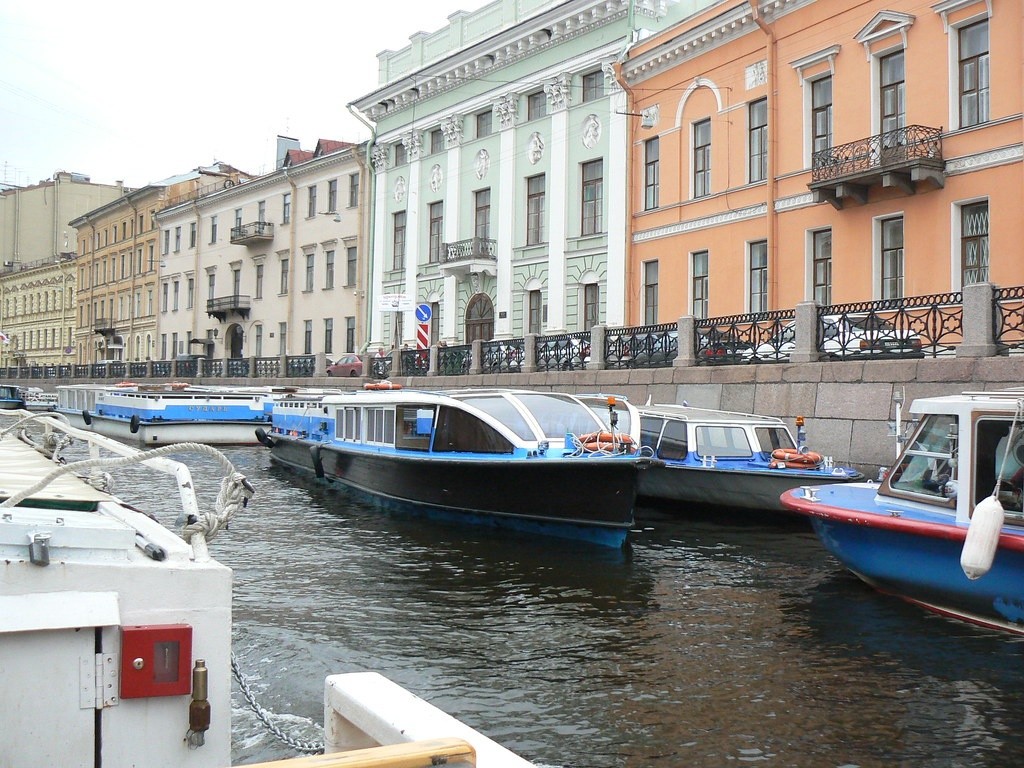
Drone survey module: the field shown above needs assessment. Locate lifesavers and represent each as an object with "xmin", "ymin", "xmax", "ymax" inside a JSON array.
[
  {"xmin": 770, "ymin": 448, "xmax": 821, "ymax": 469},
  {"xmin": 578, "ymin": 433, "xmax": 635, "ymax": 454},
  {"xmin": 165, "ymin": 383, "xmax": 190, "ymax": 388},
  {"xmin": 254, "ymin": 426, "xmax": 275, "ymax": 449},
  {"xmin": 115, "ymin": 383, "xmax": 137, "ymax": 387},
  {"xmin": 363, "ymin": 383, "xmax": 401, "ymax": 390},
  {"xmin": 82, "ymin": 409, "xmax": 91, "ymax": 426},
  {"xmin": 309, "ymin": 446, "xmax": 325, "ymax": 478},
  {"xmin": 129, "ymin": 415, "xmax": 140, "ymax": 433}
]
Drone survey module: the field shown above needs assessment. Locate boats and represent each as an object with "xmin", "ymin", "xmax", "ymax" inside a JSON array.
[
  {"xmin": 779, "ymin": 387, "xmax": 1024, "ymax": 636},
  {"xmin": 48, "ymin": 380, "xmax": 287, "ymax": 444},
  {"xmin": 253, "ymin": 381, "xmax": 668, "ymax": 550},
  {"xmin": 604, "ymin": 394, "xmax": 866, "ymax": 514},
  {"xmin": 0, "ymin": 384, "xmax": 25, "ymax": 410}
]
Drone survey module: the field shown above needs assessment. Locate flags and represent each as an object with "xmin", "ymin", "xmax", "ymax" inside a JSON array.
[{"xmin": 0, "ymin": 332, "xmax": 10, "ymax": 345}]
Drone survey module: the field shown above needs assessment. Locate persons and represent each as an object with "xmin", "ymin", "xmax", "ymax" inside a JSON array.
[
  {"xmin": 375, "ymin": 348, "xmax": 388, "ymax": 374},
  {"xmin": 436, "ymin": 341, "xmax": 446, "ymax": 347},
  {"xmin": 402, "ymin": 344, "xmax": 409, "ymax": 350}
]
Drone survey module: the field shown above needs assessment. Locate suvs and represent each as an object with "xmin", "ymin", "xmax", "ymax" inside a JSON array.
[{"xmin": 169, "ymin": 354, "xmax": 210, "ymax": 377}]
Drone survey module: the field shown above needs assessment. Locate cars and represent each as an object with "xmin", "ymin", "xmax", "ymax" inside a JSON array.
[
  {"xmin": 375, "ymin": 333, "xmax": 593, "ymax": 377},
  {"xmin": 627, "ymin": 326, "xmax": 756, "ymax": 367},
  {"xmin": 739, "ymin": 308, "xmax": 925, "ymax": 364},
  {"xmin": 326, "ymin": 353, "xmax": 364, "ymax": 378}
]
